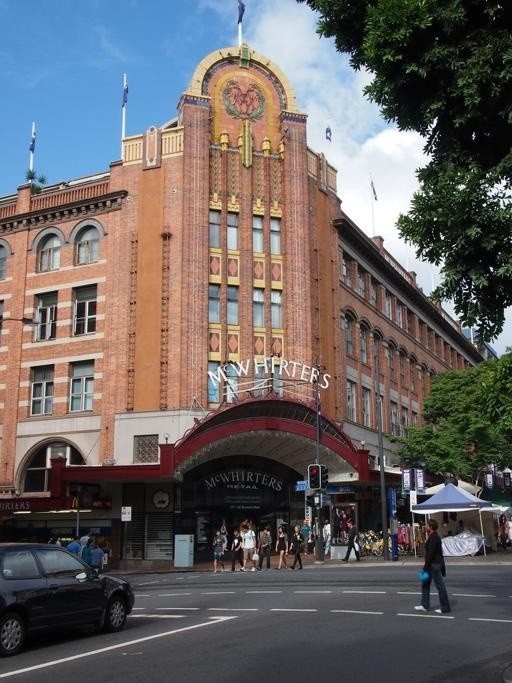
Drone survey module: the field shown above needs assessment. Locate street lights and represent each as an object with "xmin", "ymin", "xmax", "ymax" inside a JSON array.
[
  {"xmin": 442, "ymin": 557, "xmax": 445, "ymax": 577},
  {"xmin": 291, "ymin": 545, "xmax": 296, "ymax": 554},
  {"xmin": 300, "ymin": 544, "xmax": 305, "ymax": 552},
  {"xmin": 78, "ymin": 551, "xmax": 82, "ymax": 558},
  {"xmin": 260, "ymin": 550, "xmax": 268, "ymax": 557}
]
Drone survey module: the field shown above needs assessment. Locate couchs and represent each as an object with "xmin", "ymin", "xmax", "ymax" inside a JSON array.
[{"xmin": 308, "ymin": 464, "xmax": 329, "ymax": 492}]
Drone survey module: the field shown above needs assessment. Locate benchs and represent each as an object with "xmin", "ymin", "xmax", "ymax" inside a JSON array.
[
  {"xmin": 325, "ymin": 126, "xmax": 332, "ymax": 140},
  {"xmin": 29, "ymin": 130, "xmax": 38, "ymax": 153},
  {"xmin": 236, "ymin": 0, "xmax": 246, "ymax": 23},
  {"xmin": 413, "ymin": 518, "xmax": 452, "ymax": 613},
  {"xmin": 121, "ymin": 84, "xmax": 129, "ymax": 108}
]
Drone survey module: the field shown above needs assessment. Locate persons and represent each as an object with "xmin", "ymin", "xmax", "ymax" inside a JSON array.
[
  {"xmin": 342, "ymin": 518, "xmax": 362, "ymax": 562},
  {"xmin": 438, "ymin": 521, "xmax": 452, "ymax": 538},
  {"xmin": 455, "ymin": 519, "xmax": 463, "ymax": 534},
  {"xmin": 210, "ymin": 515, "xmax": 331, "ymax": 574},
  {"xmin": 397, "ymin": 520, "xmax": 426, "ymax": 556},
  {"xmin": 506, "ymin": 515, "xmax": 512, "ymax": 545},
  {"xmin": 47, "ymin": 528, "xmax": 112, "ymax": 575},
  {"xmin": 333, "ymin": 506, "xmax": 355, "ymax": 545},
  {"xmin": 497, "ymin": 513, "xmax": 508, "ymax": 552}
]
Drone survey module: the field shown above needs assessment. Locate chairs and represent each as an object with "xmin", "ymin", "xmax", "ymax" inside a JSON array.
[
  {"xmin": 252, "ymin": 554, "xmax": 259, "ymax": 561},
  {"xmin": 251, "ymin": 567, "xmax": 257, "ymax": 571},
  {"xmin": 414, "ymin": 605, "xmax": 428, "ymax": 611},
  {"xmin": 435, "ymin": 609, "xmax": 442, "ymax": 613},
  {"xmin": 343, "ymin": 559, "xmax": 348, "ymax": 562},
  {"xmin": 357, "ymin": 559, "xmax": 360, "ymax": 561},
  {"xmin": 240, "ymin": 567, "xmax": 246, "ymax": 572}
]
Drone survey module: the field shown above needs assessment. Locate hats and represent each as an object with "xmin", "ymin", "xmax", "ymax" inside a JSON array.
[{"xmin": 0, "ymin": 542, "xmax": 136, "ymax": 656}]
